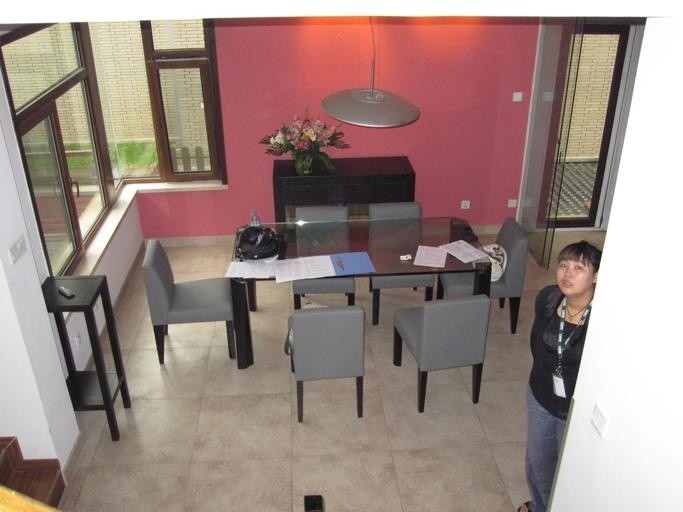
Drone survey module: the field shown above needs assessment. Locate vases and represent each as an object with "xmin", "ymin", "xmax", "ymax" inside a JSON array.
[{"xmin": 293, "ymin": 151, "xmax": 321, "ymax": 178}]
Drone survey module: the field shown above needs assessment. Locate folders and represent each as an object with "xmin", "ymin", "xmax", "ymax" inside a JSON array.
[{"xmin": 275, "ymin": 251, "xmax": 377, "ymax": 279}]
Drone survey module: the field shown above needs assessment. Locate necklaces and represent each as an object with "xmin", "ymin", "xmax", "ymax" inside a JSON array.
[{"xmin": 566, "ymin": 305, "xmax": 587, "ymax": 320}]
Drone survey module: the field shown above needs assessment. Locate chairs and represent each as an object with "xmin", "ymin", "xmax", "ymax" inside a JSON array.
[
  {"xmin": 291, "ymin": 205, "xmax": 355, "ymax": 309},
  {"xmin": 287, "ymin": 303, "xmax": 364, "ymax": 422},
  {"xmin": 437, "ymin": 217, "xmax": 528, "ymax": 334},
  {"xmin": 142, "ymin": 239, "xmax": 236, "ymax": 364},
  {"xmin": 392, "ymin": 295, "xmax": 491, "ymax": 414},
  {"xmin": 367, "ymin": 202, "xmax": 433, "ymax": 325}
]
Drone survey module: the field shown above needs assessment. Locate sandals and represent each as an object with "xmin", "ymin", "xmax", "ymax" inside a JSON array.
[{"xmin": 516, "ymin": 501, "xmax": 532, "ymax": 512}]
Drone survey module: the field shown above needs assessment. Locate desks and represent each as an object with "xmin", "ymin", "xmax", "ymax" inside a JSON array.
[
  {"xmin": 273, "ymin": 155, "xmax": 417, "ymax": 223},
  {"xmin": 40, "ymin": 274, "xmax": 131, "ymax": 442}
]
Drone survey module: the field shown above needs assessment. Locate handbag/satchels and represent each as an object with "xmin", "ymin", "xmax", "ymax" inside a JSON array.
[{"xmin": 234, "ymin": 225, "xmax": 284, "ymax": 263}]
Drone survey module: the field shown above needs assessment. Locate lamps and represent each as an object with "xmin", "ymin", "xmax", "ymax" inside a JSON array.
[{"xmin": 321, "ymin": 19, "xmax": 420, "ymax": 130}]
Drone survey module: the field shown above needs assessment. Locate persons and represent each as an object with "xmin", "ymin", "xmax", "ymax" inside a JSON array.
[{"xmin": 518, "ymin": 240, "xmax": 602, "ymax": 512}]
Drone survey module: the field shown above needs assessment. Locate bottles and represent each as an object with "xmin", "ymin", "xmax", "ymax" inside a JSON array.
[{"xmin": 249, "ymin": 209, "xmax": 260, "ymax": 227}]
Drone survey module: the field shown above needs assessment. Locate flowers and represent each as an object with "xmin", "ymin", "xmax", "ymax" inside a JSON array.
[{"xmin": 257, "ymin": 105, "xmax": 350, "ymax": 173}]
